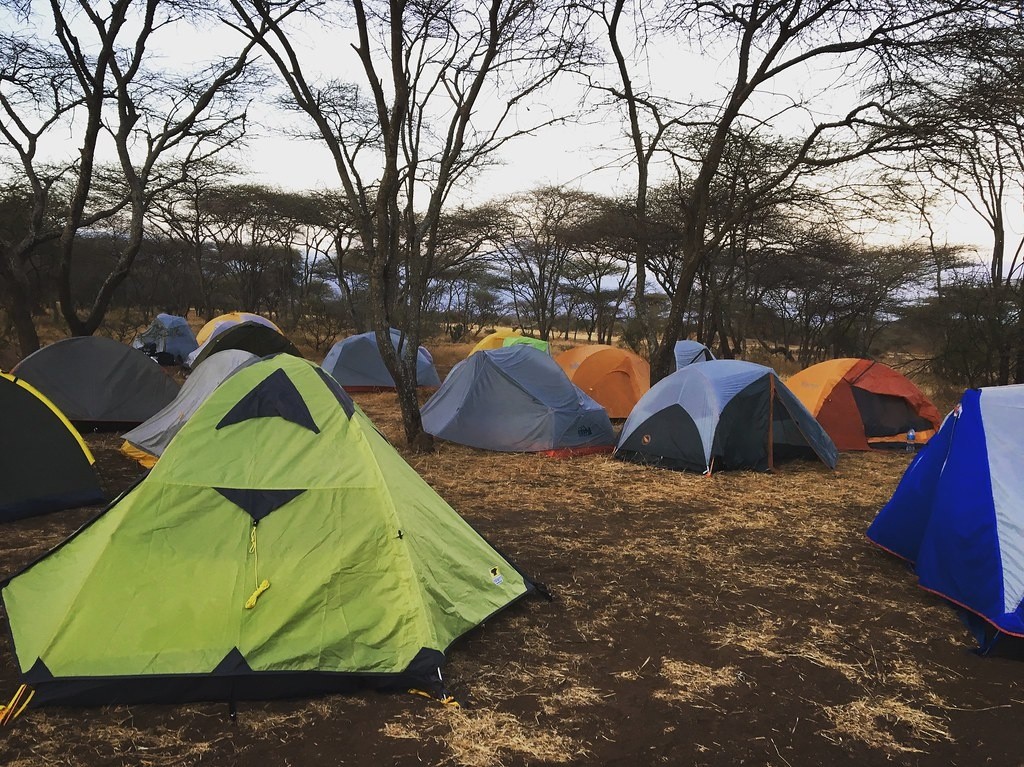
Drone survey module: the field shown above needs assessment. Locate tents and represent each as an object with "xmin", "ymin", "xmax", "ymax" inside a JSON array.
[
  {"xmin": 864, "ymin": 384, "xmax": 1024, "ymax": 662},
  {"xmin": 1, "ymin": 351, "xmax": 553, "ymax": 736},
  {"xmin": 1, "ymin": 310, "xmax": 846, "ymax": 519},
  {"xmin": 783, "ymin": 357, "xmax": 942, "ymax": 452}
]
